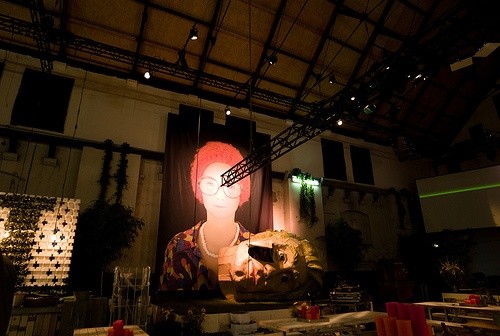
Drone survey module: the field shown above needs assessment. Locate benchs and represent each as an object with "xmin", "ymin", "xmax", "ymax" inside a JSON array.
[
  {"xmin": 426, "ymin": 319, "xmax": 463, "ymax": 336},
  {"xmin": 432, "ymin": 313, "xmax": 495, "ymax": 322},
  {"xmin": 461, "ymin": 320, "xmax": 500, "ymax": 336}
]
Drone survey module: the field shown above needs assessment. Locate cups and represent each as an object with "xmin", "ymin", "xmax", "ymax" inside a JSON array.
[
  {"xmin": 108, "ymin": 320, "xmax": 133, "ymax": 336},
  {"xmin": 385, "ymin": 302, "xmax": 399, "ymax": 320},
  {"xmin": 302, "ymin": 306, "xmax": 320, "ymax": 320},
  {"xmin": 397, "ymin": 303, "xmax": 414, "ymax": 320},
  {"xmin": 396, "ymin": 320, "xmax": 414, "ymax": 336},
  {"xmin": 383, "ymin": 316, "xmax": 398, "ymax": 336},
  {"xmin": 465, "ymin": 295, "xmax": 481, "ymax": 305},
  {"xmin": 409, "ymin": 304, "xmax": 432, "ymax": 336},
  {"xmin": 375, "ymin": 316, "xmax": 389, "ymax": 336}
]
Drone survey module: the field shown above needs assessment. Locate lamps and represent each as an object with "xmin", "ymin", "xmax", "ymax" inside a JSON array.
[{"xmin": 141, "ymin": 25, "xmax": 430, "ymax": 127}]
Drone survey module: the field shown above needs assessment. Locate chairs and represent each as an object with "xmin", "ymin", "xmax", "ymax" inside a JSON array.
[{"xmin": 442, "ymin": 292, "xmax": 481, "ymax": 321}]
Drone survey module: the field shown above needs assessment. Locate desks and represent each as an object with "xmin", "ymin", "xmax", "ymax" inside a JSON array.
[
  {"xmin": 413, "ymin": 301, "xmax": 500, "ymax": 322},
  {"xmin": 73, "ymin": 325, "xmax": 149, "ymax": 336},
  {"xmin": 258, "ymin": 311, "xmax": 388, "ymax": 332}
]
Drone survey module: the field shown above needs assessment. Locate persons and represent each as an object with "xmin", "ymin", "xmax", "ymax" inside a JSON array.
[{"xmin": 218, "ymin": 230, "xmax": 321, "ymax": 302}]
